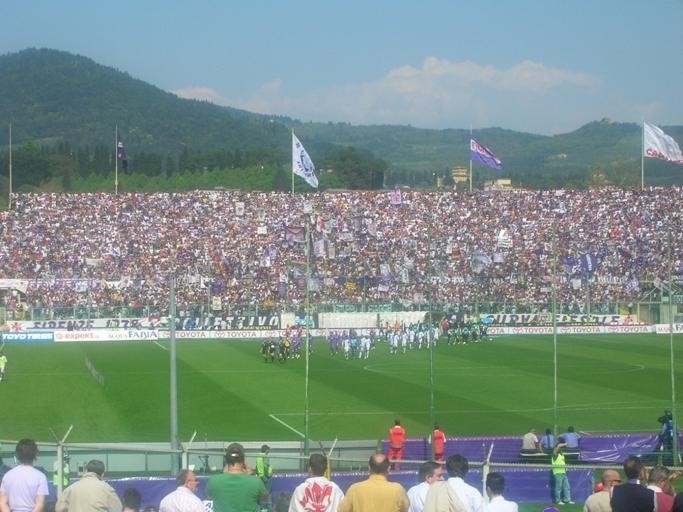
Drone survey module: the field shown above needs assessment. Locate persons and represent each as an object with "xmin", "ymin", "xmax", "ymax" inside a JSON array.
[
  {"xmin": 0, "ymin": 411, "xmax": 683, "ymax": 512},
  {"xmin": 0, "ymin": 352, "xmax": 8, "ymax": 381},
  {"xmin": 1, "ymin": 185, "xmax": 683, "ymax": 363}
]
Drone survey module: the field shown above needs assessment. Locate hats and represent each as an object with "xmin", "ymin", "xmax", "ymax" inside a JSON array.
[{"xmin": 227, "ymin": 442, "xmax": 245, "ymax": 458}]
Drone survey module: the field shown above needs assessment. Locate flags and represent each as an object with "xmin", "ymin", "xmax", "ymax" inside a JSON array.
[
  {"xmin": 643, "ymin": 119, "xmax": 683, "ymax": 164},
  {"xmin": 117, "ymin": 129, "xmax": 128, "ymax": 175},
  {"xmin": 292, "ymin": 134, "xmax": 319, "ymax": 188},
  {"xmin": 468, "ymin": 136, "xmax": 504, "ymax": 170}
]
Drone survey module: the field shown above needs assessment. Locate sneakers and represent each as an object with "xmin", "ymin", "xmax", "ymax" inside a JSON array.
[{"xmin": 555, "ymin": 501, "xmax": 577, "ymax": 505}]
